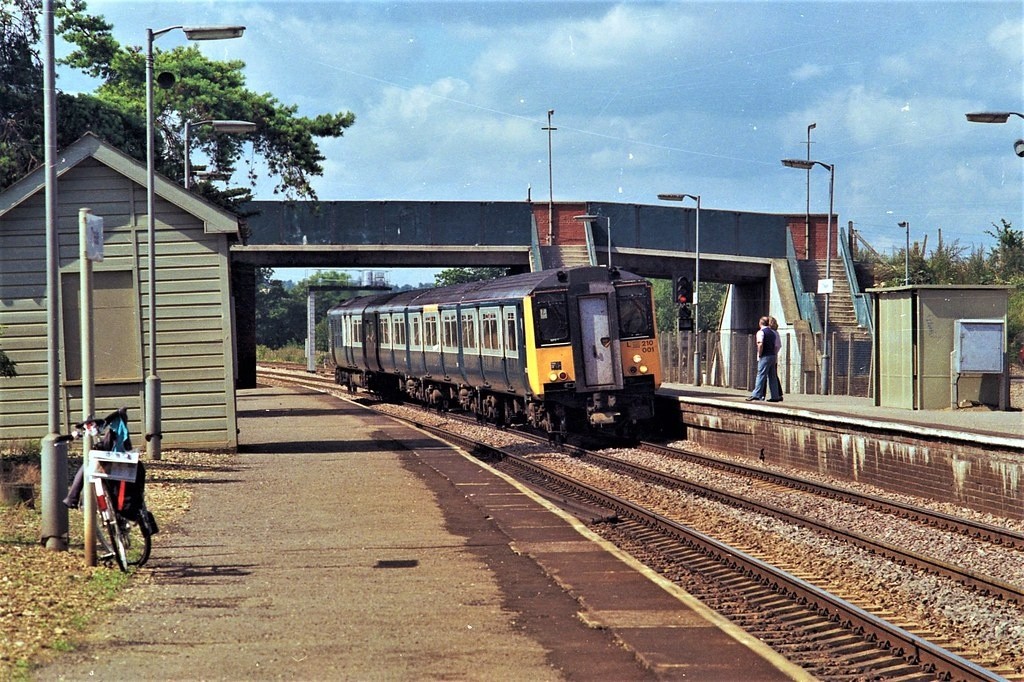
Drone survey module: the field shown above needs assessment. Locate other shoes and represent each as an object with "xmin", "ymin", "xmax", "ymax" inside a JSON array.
[
  {"xmin": 767, "ymin": 397, "xmax": 783, "ymax": 402},
  {"xmin": 744, "ymin": 395, "xmax": 765, "ymax": 401}
]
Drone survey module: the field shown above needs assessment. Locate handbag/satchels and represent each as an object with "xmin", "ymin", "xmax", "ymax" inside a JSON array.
[{"xmin": 105, "ymin": 462, "xmax": 145, "ymax": 521}]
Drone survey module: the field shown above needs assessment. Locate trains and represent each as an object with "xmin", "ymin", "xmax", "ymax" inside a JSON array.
[{"xmin": 327, "ymin": 265, "xmax": 661, "ymax": 454}]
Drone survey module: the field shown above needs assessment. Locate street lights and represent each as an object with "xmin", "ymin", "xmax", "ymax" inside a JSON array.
[
  {"xmin": 805, "ymin": 123, "xmax": 817, "ymax": 260},
  {"xmin": 183, "ymin": 119, "xmax": 257, "ymax": 192},
  {"xmin": 656, "ymin": 192, "xmax": 701, "ymax": 387},
  {"xmin": 541, "ymin": 108, "xmax": 558, "ymax": 246},
  {"xmin": 142, "ymin": 23, "xmax": 246, "ymax": 462},
  {"xmin": 897, "ymin": 221, "xmax": 910, "ymax": 286},
  {"xmin": 781, "ymin": 156, "xmax": 832, "ymax": 396}
]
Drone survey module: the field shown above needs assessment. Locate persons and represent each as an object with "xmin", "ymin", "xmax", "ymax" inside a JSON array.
[
  {"xmin": 756, "ymin": 317, "xmax": 783, "ymax": 401},
  {"xmin": 745, "ymin": 317, "xmax": 779, "ymax": 402}
]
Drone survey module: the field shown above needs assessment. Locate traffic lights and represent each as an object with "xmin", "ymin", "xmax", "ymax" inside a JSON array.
[
  {"xmin": 673, "ymin": 271, "xmax": 693, "ymax": 304},
  {"xmin": 678, "ymin": 317, "xmax": 694, "ymax": 331}
]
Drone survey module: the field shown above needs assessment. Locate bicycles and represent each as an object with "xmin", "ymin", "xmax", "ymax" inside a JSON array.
[{"xmin": 54, "ymin": 407, "xmax": 159, "ymax": 575}]
